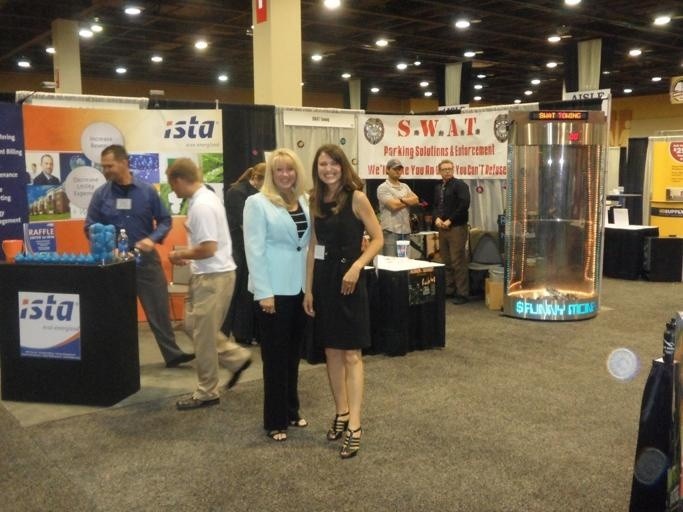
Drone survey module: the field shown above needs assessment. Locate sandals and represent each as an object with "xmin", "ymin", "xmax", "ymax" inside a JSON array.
[
  {"xmin": 289, "ymin": 417, "xmax": 307, "ymax": 429},
  {"xmin": 265, "ymin": 430, "xmax": 285, "ymax": 442}
]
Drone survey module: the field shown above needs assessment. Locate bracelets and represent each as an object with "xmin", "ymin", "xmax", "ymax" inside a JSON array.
[{"xmin": 400, "ymin": 199, "xmax": 402, "ymax": 202}]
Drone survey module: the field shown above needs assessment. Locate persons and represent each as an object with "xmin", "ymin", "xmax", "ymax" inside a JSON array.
[
  {"xmin": 33, "ymin": 155, "xmax": 60, "ymax": 184},
  {"xmin": 165, "ymin": 159, "xmax": 252, "ymax": 408},
  {"xmin": 84, "ymin": 145, "xmax": 194, "ymax": 367},
  {"xmin": 376, "ymin": 159, "xmax": 419, "ymax": 256},
  {"xmin": 220, "ymin": 163, "xmax": 267, "ymax": 346},
  {"xmin": 432, "ymin": 160, "xmax": 470, "ymax": 304},
  {"xmin": 244, "ymin": 147, "xmax": 312, "ymax": 441},
  {"xmin": 303, "ymin": 144, "xmax": 385, "ymax": 458}
]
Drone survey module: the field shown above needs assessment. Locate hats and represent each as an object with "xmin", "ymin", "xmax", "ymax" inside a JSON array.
[{"xmin": 387, "ymin": 160, "xmax": 403, "ymax": 170}]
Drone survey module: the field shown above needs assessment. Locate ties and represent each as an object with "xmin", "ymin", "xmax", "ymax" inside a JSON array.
[{"xmin": 439, "ymin": 185, "xmax": 444, "ymax": 218}]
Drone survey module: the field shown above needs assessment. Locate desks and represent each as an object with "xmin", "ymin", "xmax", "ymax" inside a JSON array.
[
  {"xmin": 604, "ymin": 224, "xmax": 659, "ymax": 280},
  {"xmin": 0, "ymin": 258, "xmax": 141, "ymax": 407},
  {"xmin": 302, "ymin": 255, "xmax": 446, "ymax": 365},
  {"xmin": 641, "ymin": 237, "xmax": 683, "ymax": 282}
]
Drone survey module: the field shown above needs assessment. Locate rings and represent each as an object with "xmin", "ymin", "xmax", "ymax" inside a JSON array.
[{"xmin": 262, "ymin": 310, "xmax": 265, "ymax": 312}]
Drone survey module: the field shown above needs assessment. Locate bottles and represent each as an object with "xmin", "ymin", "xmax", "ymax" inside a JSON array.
[
  {"xmin": 116, "ymin": 228, "xmax": 129, "ymax": 261},
  {"xmin": 659, "ymin": 316, "xmax": 677, "ymax": 365}
]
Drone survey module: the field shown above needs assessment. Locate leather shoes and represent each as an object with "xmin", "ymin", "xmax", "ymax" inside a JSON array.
[
  {"xmin": 225, "ymin": 358, "xmax": 252, "ymax": 388},
  {"xmin": 177, "ymin": 397, "xmax": 220, "ymax": 409},
  {"xmin": 165, "ymin": 353, "xmax": 195, "ymax": 367},
  {"xmin": 452, "ymin": 295, "xmax": 467, "ymax": 304}
]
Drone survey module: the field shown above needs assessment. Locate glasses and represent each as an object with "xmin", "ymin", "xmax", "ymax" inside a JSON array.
[{"xmin": 439, "ymin": 167, "xmax": 452, "ymax": 170}]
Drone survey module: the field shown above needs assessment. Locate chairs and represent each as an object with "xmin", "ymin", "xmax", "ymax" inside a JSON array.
[
  {"xmin": 466, "ymin": 228, "xmax": 503, "ymax": 297},
  {"xmin": 167, "ymin": 245, "xmax": 191, "ymax": 330}
]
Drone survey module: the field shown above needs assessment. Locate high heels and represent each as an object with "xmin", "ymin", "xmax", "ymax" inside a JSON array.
[
  {"xmin": 326, "ymin": 413, "xmax": 348, "ymax": 440},
  {"xmin": 339, "ymin": 429, "xmax": 362, "ymax": 458}
]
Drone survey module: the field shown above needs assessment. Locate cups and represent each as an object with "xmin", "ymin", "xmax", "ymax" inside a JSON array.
[
  {"xmin": 395, "ymin": 240, "xmax": 410, "ymax": 257},
  {"xmin": 617, "ymin": 186, "xmax": 624, "ymax": 194},
  {"xmin": 487, "ymin": 266, "xmax": 504, "ymax": 281},
  {"xmin": 2, "ymin": 239, "xmax": 22, "ymax": 264}
]
